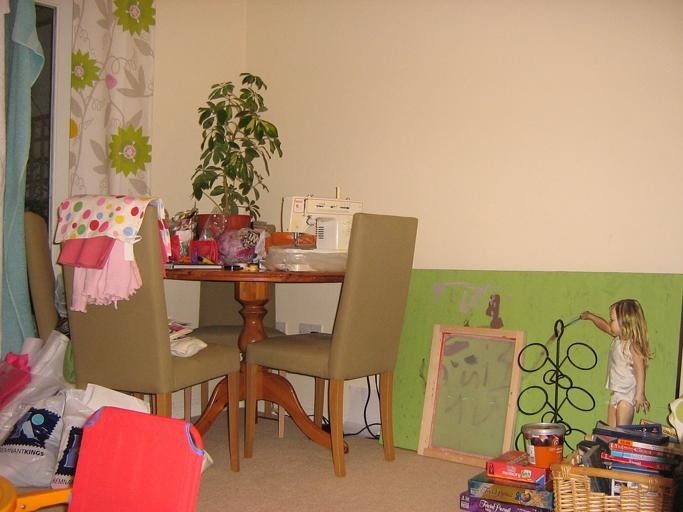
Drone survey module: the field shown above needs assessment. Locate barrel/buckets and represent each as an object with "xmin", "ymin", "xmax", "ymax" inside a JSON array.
[{"xmin": 521, "ymin": 421, "xmax": 567, "ymax": 465}]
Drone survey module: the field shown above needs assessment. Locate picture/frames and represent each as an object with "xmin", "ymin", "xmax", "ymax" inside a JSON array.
[{"xmin": 417, "ymin": 323, "xmax": 527, "ymax": 468}]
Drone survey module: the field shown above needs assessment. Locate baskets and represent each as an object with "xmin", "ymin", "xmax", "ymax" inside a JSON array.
[{"xmin": 551, "ymin": 444, "xmax": 683, "ymax": 512}]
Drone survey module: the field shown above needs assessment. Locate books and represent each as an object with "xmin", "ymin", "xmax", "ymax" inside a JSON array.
[
  {"xmin": 166, "ymin": 318, "xmax": 192, "ymax": 340},
  {"xmin": 163, "ymin": 263, "xmax": 224, "ymax": 271},
  {"xmin": 458, "ymin": 420, "xmax": 681, "ymax": 512}
]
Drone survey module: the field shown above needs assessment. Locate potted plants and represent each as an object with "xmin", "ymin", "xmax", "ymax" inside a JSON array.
[{"xmin": 189, "ymin": 72, "xmax": 283, "ymax": 243}]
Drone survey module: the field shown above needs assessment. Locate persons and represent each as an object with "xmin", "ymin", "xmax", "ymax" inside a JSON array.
[{"xmin": 579, "ymin": 298, "xmax": 656, "ymax": 429}]
